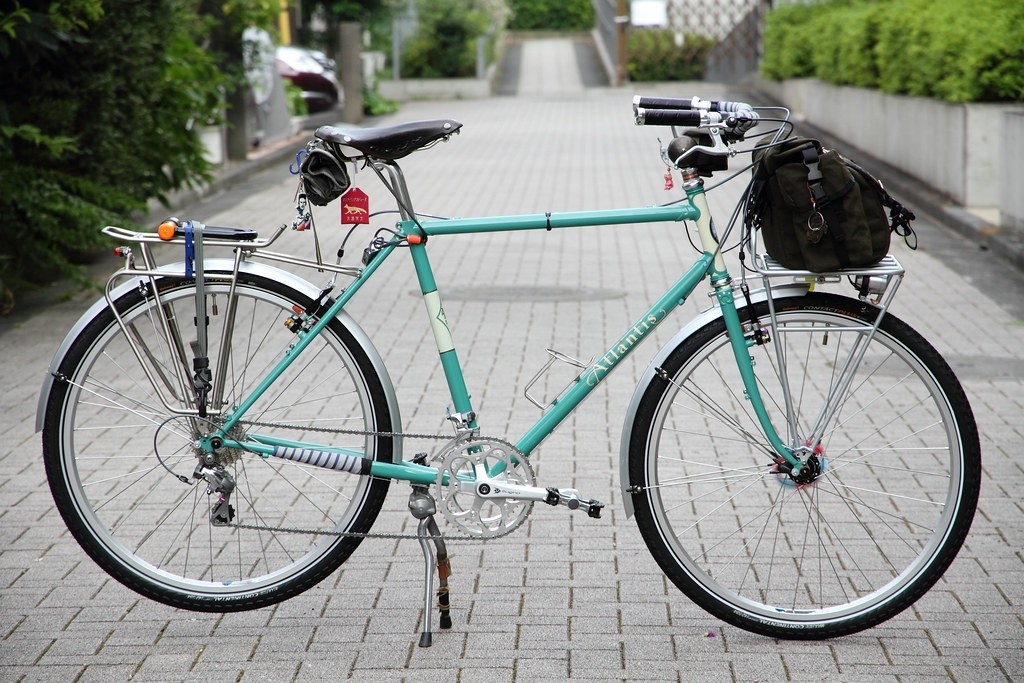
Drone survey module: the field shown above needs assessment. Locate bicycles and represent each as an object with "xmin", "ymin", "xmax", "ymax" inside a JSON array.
[{"xmin": 35, "ymin": 95, "xmax": 981, "ymax": 648}]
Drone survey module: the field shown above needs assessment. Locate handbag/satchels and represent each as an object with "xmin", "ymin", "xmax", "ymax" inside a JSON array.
[{"xmin": 752, "ymin": 131, "xmax": 917, "ymax": 273}]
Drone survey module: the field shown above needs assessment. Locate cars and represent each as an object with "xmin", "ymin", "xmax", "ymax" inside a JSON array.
[{"xmin": 274, "ymin": 45, "xmax": 344, "ymax": 115}]
